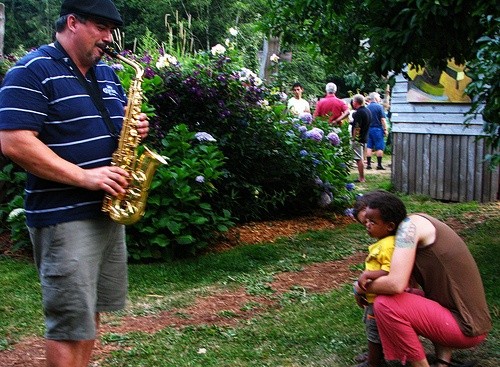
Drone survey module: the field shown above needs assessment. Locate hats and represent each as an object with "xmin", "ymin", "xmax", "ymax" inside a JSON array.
[{"xmin": 60, "ymin": 0, "xmax": 123, "ymax": 27}]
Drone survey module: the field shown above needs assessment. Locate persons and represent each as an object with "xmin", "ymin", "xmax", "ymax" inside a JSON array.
[
  {"xmin": 288, "ymin": 83, "xmax": 491, "ymax": 367},
  {"xmin": 0, "ymin": 1, "xmax": 150, "ymax": 366}
]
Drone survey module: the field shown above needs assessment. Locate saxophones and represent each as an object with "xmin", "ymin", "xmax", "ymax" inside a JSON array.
[{"xmin": 98, "ymin": 44, "xmax": 168, "ymax": 224}]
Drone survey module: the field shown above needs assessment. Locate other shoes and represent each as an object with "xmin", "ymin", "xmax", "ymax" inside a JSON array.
[
  {"xmin": 367, "ymin": 166, "xmax": 372, "ymax": 169},
  {"xmin": 358, "ymin": 178, "xmax": 365, "ymax": 182},
  {"xmin": 377, "ymin": 166, "xmax": 385, "ymax": 170}
]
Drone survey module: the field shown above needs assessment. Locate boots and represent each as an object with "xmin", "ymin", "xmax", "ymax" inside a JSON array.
[{"xmin": 355, "ymin": 340, "xmax": 386, "ymax": 367}]
